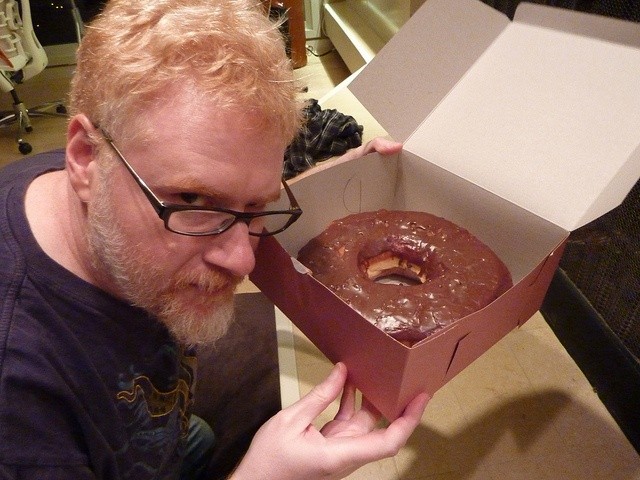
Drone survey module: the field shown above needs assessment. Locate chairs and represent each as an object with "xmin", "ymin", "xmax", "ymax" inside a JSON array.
[{"xmin": 0, "ymin": 0, "xmax": 67, "ymax": 155}]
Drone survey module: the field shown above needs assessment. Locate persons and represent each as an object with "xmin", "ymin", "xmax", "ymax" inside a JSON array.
[{"xmin": 0, "ymin": 0, "xmax": 431, "ymax": 480}]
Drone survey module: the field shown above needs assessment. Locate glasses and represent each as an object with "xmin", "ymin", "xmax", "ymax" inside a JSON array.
[{"xmin": 100, "ymin": 127, "xmax": 303, "ymax": 238}]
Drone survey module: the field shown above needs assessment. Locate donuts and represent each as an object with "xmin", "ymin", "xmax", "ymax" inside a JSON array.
[{"xmin": 295, "ymin": 207, "xmax": 515, "ymax": 346}]
